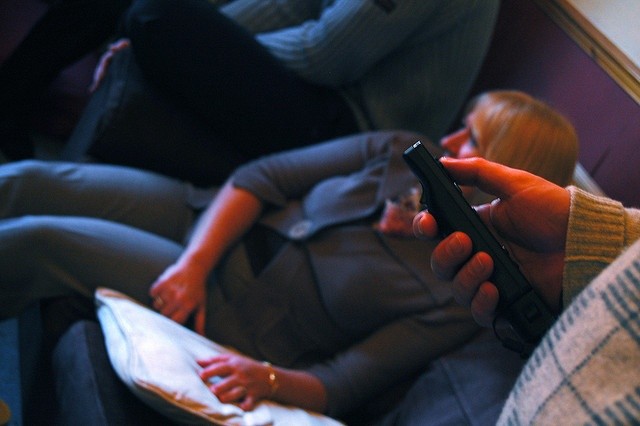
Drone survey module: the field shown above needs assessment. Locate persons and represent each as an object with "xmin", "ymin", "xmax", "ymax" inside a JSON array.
[
  {"xmin": 1, "ymin": 90, "xmax": 578, "ymax": 414},
  {"xmin": 412, "ymin": 155, "xmax": 639, "ymax": 426},
  {"xmin": 61, "ymin": 0, "xmax": 501, "ymax": 188}
]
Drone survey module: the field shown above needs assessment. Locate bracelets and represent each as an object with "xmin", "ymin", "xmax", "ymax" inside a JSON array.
[{"xmin": 263, "ymin": 362, "xmax": 279, "ymax": 400}]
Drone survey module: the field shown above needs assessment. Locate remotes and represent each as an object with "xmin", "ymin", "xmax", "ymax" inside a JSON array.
[{"xmin": 403, "ymin": 139, "xmax": 557, "ymax": 344}]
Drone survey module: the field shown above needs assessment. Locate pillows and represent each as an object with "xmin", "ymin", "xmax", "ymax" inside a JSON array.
[{"xmin": 92, "ymin": 285, "xmax": 358, "ymax": 426}]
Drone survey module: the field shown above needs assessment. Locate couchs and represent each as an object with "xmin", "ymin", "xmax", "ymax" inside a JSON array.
[{"xmin": 52, "ymin": 317, "xmax": 525, "ymax": 426}]
